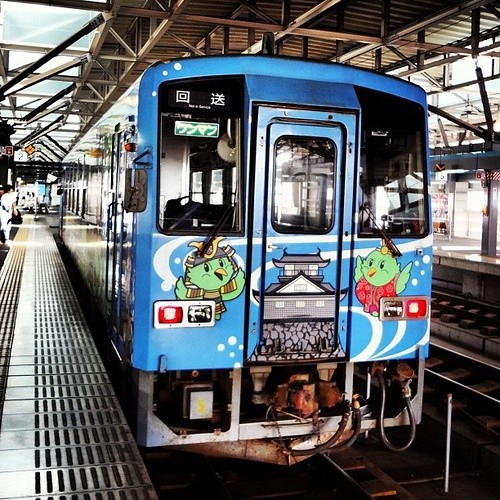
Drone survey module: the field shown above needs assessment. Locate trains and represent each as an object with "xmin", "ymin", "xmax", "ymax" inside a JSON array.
[{"xmin": 43, "ymin": 34, "xmax": 439, "ymax": 473}]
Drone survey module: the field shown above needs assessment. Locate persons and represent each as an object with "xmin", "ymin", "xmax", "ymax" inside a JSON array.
[
  {"xmin": 0, "ymin": 186, "xmax": 19, "ymax": 245},
  {"xmin": 15, "ymin": 188, "xmax": 49, "ymax": 214},
  {"xmin": 0, "ymin": 189, "xmax": 10, "ymax": 252}
]
